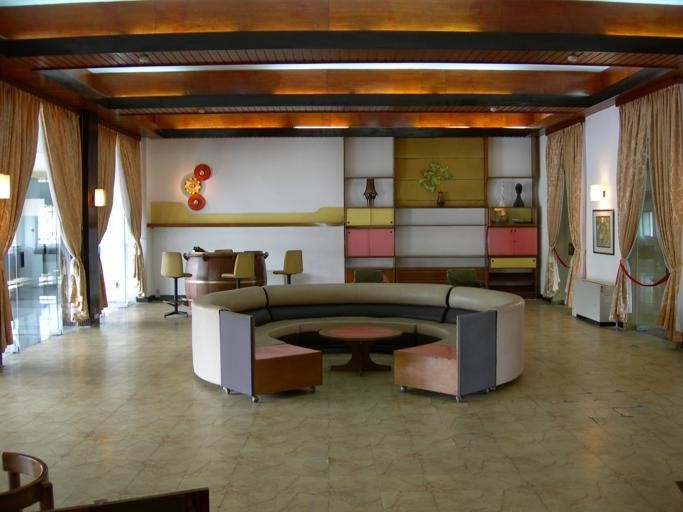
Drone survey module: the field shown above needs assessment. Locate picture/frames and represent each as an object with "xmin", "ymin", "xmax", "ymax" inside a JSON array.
[{"xmin": 592, "ymin": 208, "xmax": 615, "ymax": 256}]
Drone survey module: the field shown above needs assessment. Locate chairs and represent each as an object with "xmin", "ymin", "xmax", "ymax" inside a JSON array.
[
  {"xmin": 353, "ymin": 268, "xmax": 385, "ymax": 284},
  {"xmin": 446, "ymin": 269, "xmax": 484, "ymax": 288},
  {"xmin": 215, "ymin": 249, "xmax": 233, "ymax": 254},
  {"xmin": 162, "ymin": 251, "xmax": 192, "ymax": 318},
  {"xmin": 0, "ymin": 453, "xmax": 54, "ymax": 512},
  {"xmin": 221, "ymin": 251, "xmax": 255, "ymax": 289},
  {"xmin": 273, "ymin": 250, "xmax": 303, "ymax": 283}
]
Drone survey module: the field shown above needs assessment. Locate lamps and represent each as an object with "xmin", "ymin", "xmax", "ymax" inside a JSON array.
[
  {"xmin": 0, "ymin": 172, "xmax": 11, "ymax": 202},
  {"xmin": 92, "ymin": 188, "xmax": 107, "ymax": 207},
  {"xmin": 589, "ymin": 183, "xmax": 610, "ymax": 206}
]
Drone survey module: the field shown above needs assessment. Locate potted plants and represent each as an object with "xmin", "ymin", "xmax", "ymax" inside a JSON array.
[{"xmin": 416, "ymin": 161, "xmax": 455, "ymax": 207}]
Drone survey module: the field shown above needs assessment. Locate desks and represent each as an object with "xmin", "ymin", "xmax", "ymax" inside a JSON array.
[{"xmin": 186, "ymin": 251, "xmax": 267, "ymax": 308}]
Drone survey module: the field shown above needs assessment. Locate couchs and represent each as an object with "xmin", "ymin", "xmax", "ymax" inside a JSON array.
[
  {"xmin": 262, "ymin": 283, "xmax": 454, "ymax": 355},
  {"xmin": 192, "ymin": 286, "xmax": 323, "ymax": 404},
  {"xmin": 394, "ymin": 287, "xmax": 527, "ymax": 402}
]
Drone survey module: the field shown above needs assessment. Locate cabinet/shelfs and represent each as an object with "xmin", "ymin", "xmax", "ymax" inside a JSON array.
[
  {"xmin": 571, "ymin": 280, "xmax": 617, "ymax": 325},
  {"xmin": 394, "ymin": 135, "xmax": 486, "ymax": 289},
  {"xmin": 343, "ymin": 133, "xmax": 394, "ymax": 284},
  {"xmin": 487, "ymin": 133, "xmax": 541, "ymax": 299}
]
